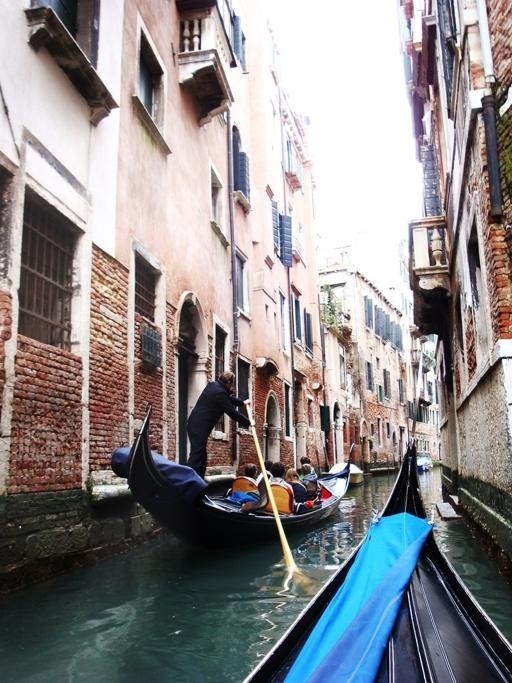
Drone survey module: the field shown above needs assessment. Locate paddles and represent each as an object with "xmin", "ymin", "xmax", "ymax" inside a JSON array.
[{"xmin": 244, "ymin": 401, "xmax": 310, "ymax": 567}]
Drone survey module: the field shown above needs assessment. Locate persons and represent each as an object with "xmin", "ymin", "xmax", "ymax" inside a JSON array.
[
  {"xmin": 184, "ymin": 368, "xmax": 258, "ymax": 481},
  {"xmin": 264, "ymin": 460, "xmax": 273, "ymax": 473},
  {"xmin": 284, "ymin": 467, "xmax": 310, "ymax": 501},
  {"xmin": 242, "ymin": 462, "xmax": 259, "ymax": 480},
  {"xmin": 296, "ymin": 456, "xmax": 315, "ymax": 478},
  {"xmin": 266, "ymin": 461, "xmax": 303, "ymax": 513},
  {"xmin": 300, "ymin": 463, "xmax": 319, "ymax": 494}
]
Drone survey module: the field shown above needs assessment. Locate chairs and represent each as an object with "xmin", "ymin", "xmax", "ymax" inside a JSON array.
[
  {"xmin": 264, "ymin": 482, "xmax": 294, "ymax": 515},
  {"xmin": 231, "ymin": 476, "xmax": 259, "ymax": 494}
]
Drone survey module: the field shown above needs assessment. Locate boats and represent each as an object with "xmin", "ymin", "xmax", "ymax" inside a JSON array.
[
  {"xmin": 320, "ymin": 464, "xmax": 364, "ymax": 486},
  {"xmin": 126, "ymin": 404, "xmax": 355, "ymax": 543},
  {"xmin": 242, "ymin": 440, "xmax": 512, "ymax": 683},
  {"xmin": 413, "ymin": 450, "xmax": 433, "ymax": 470}
]
